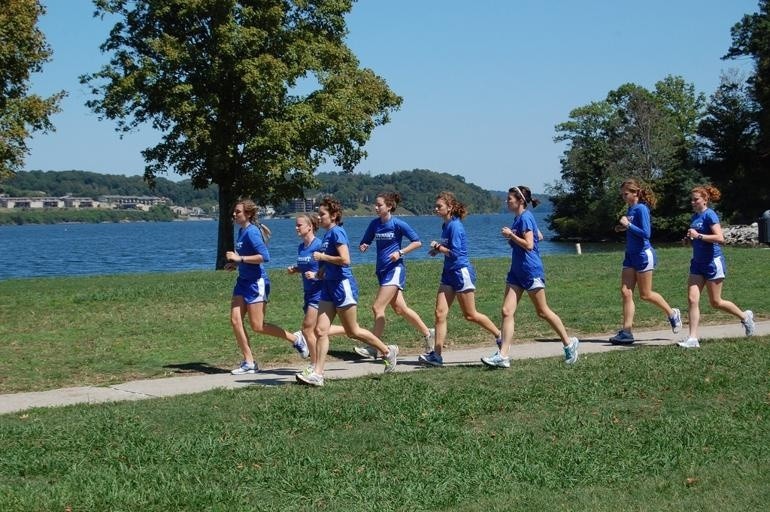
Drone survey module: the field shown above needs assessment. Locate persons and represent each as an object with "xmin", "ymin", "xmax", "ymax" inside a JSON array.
[
  {"xmin": 481, "ymin": 186, "xmax": 578, "ymax": 368},
  {"xmin": 295, "ymin": 196, "xmax": 399, "ymax": 388},
  {"xmin": 609, "ymin": 179, "xmax": 682, "ymax": 345},
  {"xmin": 287, "ymin": 212, "xmax": 346, "ymax": 376},
  {"xmin": 675, "ymin": 185, "xmax": 754, "ymax": 348},
  {"xmin": 353, "ymin": 191, "xmax": 436, "ymax": 359},
  {"xmin": 418, "ymin": 191, "xmax": 510, "ymax": 367},
  {"xmin": 223, "ymin": 199, "xmax": 310, "ymax": 375}
]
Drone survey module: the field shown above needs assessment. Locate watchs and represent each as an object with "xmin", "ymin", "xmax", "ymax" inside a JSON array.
[
  {"xmin": 698, "ymin": 234, "xmax": 703, "ymax": 240},
  {"xmin": 398, "ymin": 250, "xmax": 404, "ymax": 258}
]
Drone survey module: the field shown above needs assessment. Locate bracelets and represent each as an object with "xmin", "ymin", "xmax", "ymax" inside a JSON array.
[
  {"xmin": 434, "ymin": 242, "xmax": 443, "ymax": 251},
  {"xmin": 508, "ymin": 233, "xmax": 513, "ymax": 238},
  {"xmin": 241, "ymin": 256, "xmax": 243, "ymax": 263},
  {"xmin": 626, "ymin": 222, "xmax": 631, "ymax": 230}
]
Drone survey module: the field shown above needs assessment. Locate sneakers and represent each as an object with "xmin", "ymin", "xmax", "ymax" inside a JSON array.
[
  {"xmin": 231, "ymin": 361, "xmax": 259, "ymax": 374},
  {"xmin": 419, "ymin": 352, "xmax": 443, "ymax": 367},
  {"xmin": 296, "ymin": 366, "xmax": 324, "ymax": 387},
  {"xmin": 741, "ymin": 310, "xmax": 755, "ymax": 337},
  {"xmin": 384, "ymin": 344, "xmax": 399, "ymax": 373},
  {"xmin": 564, "ymin": 337, "xmax": 579, "ymax": 365},
  {"xmin": 353, "ymin": 346, "xmax": 383, "ymax": 358},
  {"xmin": 609, "ymin": 330, "xmax": 635, "ymax": 345},
  {"xmin": 293, "ymin": 331, "xmax": 310, "ymax": 359},
  {"xmin": 425, "ymin": 329, "xmax": 436, "ymax": 354},
  {"xmin": 481, "ymin": 352, "xmax": 511, "ymax": 368},
  {"xmin": 667, "ymin": 308, "xmax": 682, "ymax": 334},
  {"xmin": 676, "ymin": 338, "xmax": 701, "ymax": 348}
]
